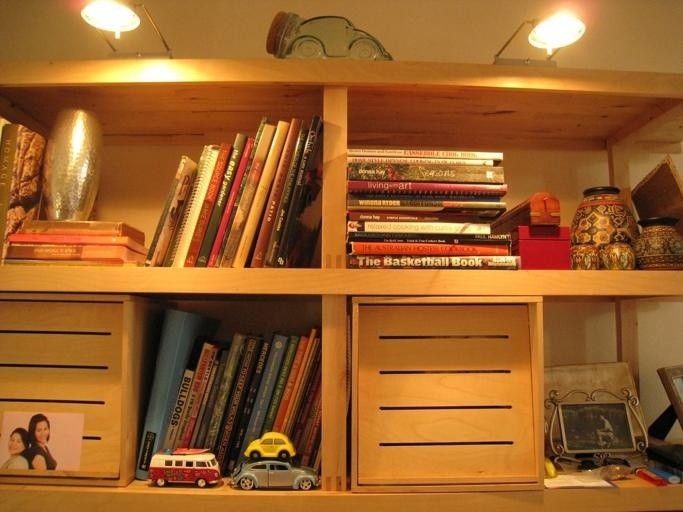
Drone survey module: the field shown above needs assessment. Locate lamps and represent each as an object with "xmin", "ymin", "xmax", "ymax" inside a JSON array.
[
  {"xmin": 486, "ymin": 7, "xmax": 589, "ymax": 67},
  {"xmin": 78, "ymin": 1, "xmax": 174, "ymax": 59}
]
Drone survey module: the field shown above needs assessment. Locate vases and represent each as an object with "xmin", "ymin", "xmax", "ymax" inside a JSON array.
[{"xmin": 565, "ymin": 185, "xmax": 681, "ymax": 270}]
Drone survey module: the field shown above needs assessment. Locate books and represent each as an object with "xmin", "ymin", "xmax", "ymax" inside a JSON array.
[
  {"xmin": 135, "ymin": 309, "xmax": 322, "ymax": 482},
  {"xmin": 145, "ymin": 115, "xmax": 324, "ymax": 269},
  {"xmin": 346, "ymin": 144, "xmax": 522, "ymax": 270},
  {"xmin": 4, "ymin": 218, "xmax": 149, "ymax": 270},
  {"xmin": 1, "ymin": 117, "xmax": 47, "ymax": 263}
]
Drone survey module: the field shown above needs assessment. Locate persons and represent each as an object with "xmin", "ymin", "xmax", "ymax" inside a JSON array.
[
  {"xmin": 28, "ymin": 414, "xmax": 57, "ymax": 470},
  {"xmin": 2, "ymin": 428, "xmax": 31, "ymax": 470},
  {"xmin": 595, "ymin": 414, "xmax": 614, "ymax": 448}
]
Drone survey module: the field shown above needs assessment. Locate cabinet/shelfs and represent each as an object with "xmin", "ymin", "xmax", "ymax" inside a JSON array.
[
  {"xmin": 336, "ymin": 51, "xmax": 682, "ymax": 512},
  {"xmin": 0, "ymin": 56, "xmax": 336, "ymax": 511}
]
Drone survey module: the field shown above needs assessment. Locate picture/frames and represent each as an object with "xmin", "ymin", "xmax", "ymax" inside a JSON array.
[
  {"xmin": 543, "ymin": 383, "xmax": 653, "ymax": 467},
  {"xmin": 646, "ymin": 357, "xmax": 683, "ymax": 447}
]
